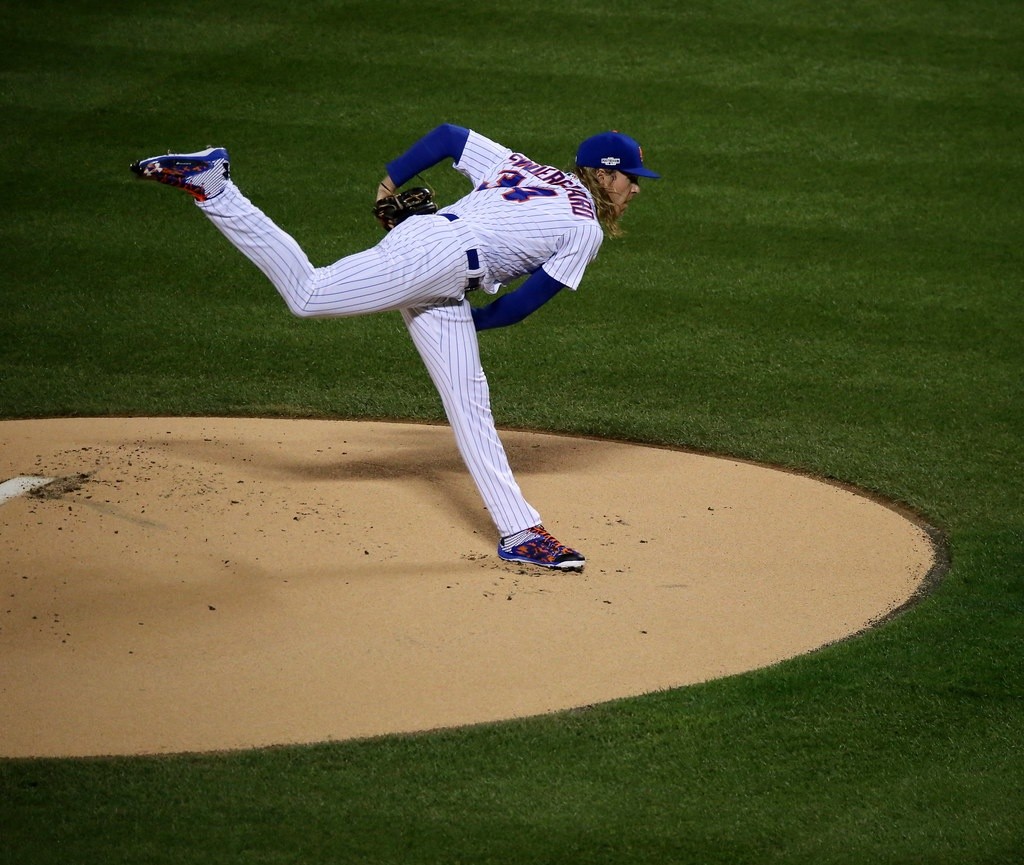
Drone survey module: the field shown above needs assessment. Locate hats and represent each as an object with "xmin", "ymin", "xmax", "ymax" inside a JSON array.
[{"xmin": 574, "ymin": 129, "xmax": 661, "ymax": 179}]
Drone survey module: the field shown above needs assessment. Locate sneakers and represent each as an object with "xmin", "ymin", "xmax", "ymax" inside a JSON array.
[
  {"xmin": 128, "ymin": 146, "xmax": 231, "ymax": 204},
  {"xmin": 497, "ymin": 524, "xmax": 586, "ymax": 573}
]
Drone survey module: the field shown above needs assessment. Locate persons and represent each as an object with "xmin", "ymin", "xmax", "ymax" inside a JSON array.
[{"xmin": 129, "ymin": 122, "xmax": 661, "ymax": 569}]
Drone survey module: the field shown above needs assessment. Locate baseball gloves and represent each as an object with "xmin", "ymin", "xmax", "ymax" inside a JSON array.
[{"xmin": 369, "ymin": 185, "xmax": 440, "ymax": 234}]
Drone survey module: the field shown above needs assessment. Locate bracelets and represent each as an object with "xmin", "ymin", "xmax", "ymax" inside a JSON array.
[{"xmin": 379, "ymin": 181, "xmax": 394, "ymax": 196}]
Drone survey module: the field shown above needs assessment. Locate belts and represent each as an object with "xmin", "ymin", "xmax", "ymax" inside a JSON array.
[{"xmin": 435, "ymin": 213, "xmax": 481, "ymax": 293}]
